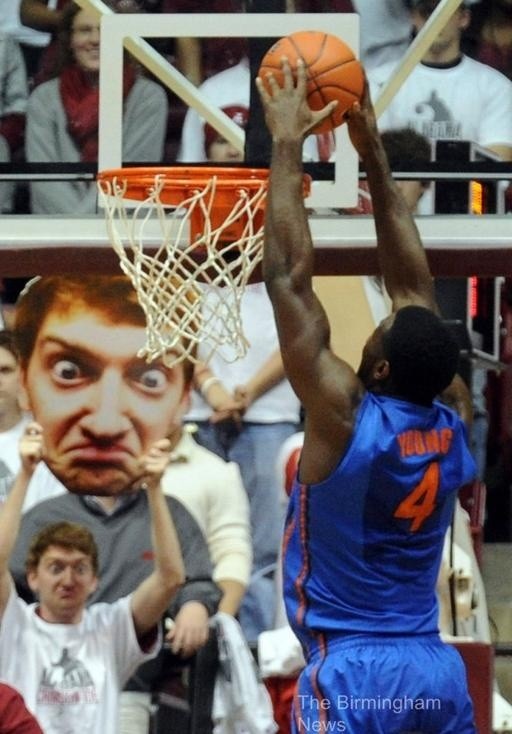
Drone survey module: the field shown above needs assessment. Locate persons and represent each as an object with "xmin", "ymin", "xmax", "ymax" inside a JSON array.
[{"xmin": 0, "ymin": 0, "xmax": 511, "ymax": 734}]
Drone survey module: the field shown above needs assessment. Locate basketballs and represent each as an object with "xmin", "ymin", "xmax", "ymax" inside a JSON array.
[{"xmin": 259, "ymin": 32, "xmax": 365, "ymax": 134}]
